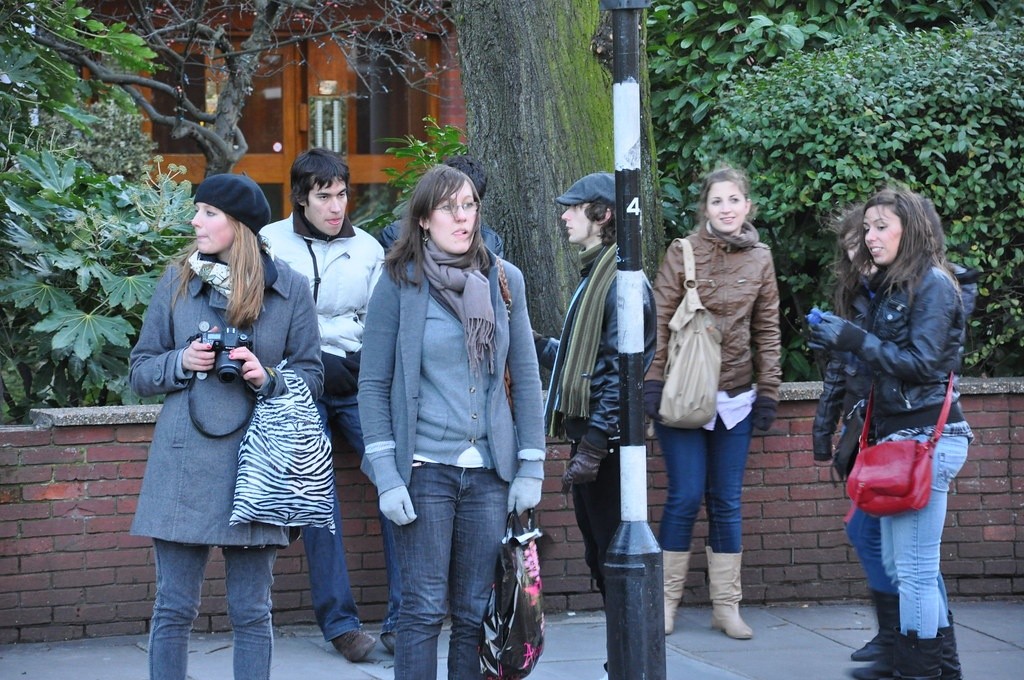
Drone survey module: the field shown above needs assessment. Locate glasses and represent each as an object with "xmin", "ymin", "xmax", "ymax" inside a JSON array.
[{"xmin": 432, "ymin": 202, "xmax": 481, "ymax": 213}]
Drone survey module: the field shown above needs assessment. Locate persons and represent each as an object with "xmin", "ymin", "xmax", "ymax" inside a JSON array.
[
  {"xmin": 355, "ymin": 165, "xmax": 547, "ymax": 679},
  {"xmin": 256, "ymin": 149, "xmax": 393, "ymax": 663},
  {"xmin": 805, "ymin": 189, "xmax": 973, "ymax": 680},
  {"xmin": 644, "ymin": 167, "xmax": 780, "ymax": 640},
  {"xmin": 126, "ymin": 173, "xmax": 323, "ymax": 678},
  {"xmin": 527, "ymin": 171, "xmax": 656, "ymax": 671},
  {"xmin": 380, "ymin": 158, "xmax": 506, "ymax": 266}
]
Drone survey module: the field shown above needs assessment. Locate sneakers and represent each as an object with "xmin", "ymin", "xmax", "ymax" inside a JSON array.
[
  {"xmin": 332, "ymin": 631, "xmax": 376, "ymax": 662},
  {"xmin": 381, "ymin": 633, "xmax": 395, "ymax": 652}
]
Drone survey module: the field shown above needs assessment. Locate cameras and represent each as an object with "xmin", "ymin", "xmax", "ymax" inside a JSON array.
[{"xmin": 202, "ymin": 327, "xmax": 254, "ymax": 384}]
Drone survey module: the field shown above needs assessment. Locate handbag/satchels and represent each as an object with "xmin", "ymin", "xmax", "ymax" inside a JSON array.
[
  {"xmin": 229, "ymin": 370, "xmax": 335, "ymax": 535},
  {"xmin": 846, "ymin": 440, "xmax": 934, "ymax": 516},
  {"xmin": 480, "ymin": 507, "xmax": 545, "ymax": 680},
  {"xmin": 658, "ymin": 239, "xmax": 723, "ymax": 427}
]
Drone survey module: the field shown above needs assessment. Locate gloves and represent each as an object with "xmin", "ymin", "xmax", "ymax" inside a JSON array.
[
  {"xmin": 807, "ymin": 314, "xmax": 848, "ymax": 351},
  {"xmin": 321, "ymin": 352, "xmax": 361, "ymax": 397},
  {"xmin": 379, "ymin": 485, "xmax": 417, "ymax": 526},
  {"xmin": 644, "ymin": 381, "xmax": 664, "ymax": 418},
  {"xmin": 507, "ymin": 477, "xmax": 543, "ymax": 516},
  {"xmin": 562, "ymin": 435, "xmax": 608, "ymax": 485},
  {"xmin": 754, "ymin": 397, "xmax": 776, "ymax": 431}
]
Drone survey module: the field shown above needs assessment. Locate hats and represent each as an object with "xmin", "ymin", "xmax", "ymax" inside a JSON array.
[
  {"xmin": 194, "ymin": 170, "xmax": 272, "ymax": 234},
  {"xmin": 556, "ymin": 170, "xmax": 617, "ymax": 205}
]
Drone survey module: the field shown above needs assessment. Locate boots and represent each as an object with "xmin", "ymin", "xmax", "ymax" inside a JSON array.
[
  {"xmin": 852, "ymin": 640, "xmax": 894, "ymax": 680},
  {"xmin": 893, "ymin": 626, "xmax": 945, "ymax": 680},
  {"xmin": 705, "ymin": 546, "xmax": 753, "ymax": 638},
  {"xmin": 938, "ymin": 627, "xmax": 961, "ymax": 680},
  {"xmin": 851, "ymin": 591, "xmax": 900, "ymax": 661},
  {"xmin": 663, "ymin": 550, "xmax": 690, "ymax": 635}
]
